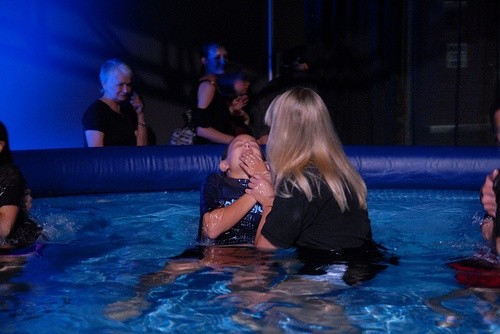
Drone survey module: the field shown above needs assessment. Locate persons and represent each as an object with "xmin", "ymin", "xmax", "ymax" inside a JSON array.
[
  {"xmin": 480, "ymin": 107, "xmax": 500, "ymax": 254},
  {"xmin": 182, "ymin": 37, "xmax": 257, "ymax": 144},
  {"xmin": 244, "ymin": 86, "xmax": 372, "ymax": 250},
  {"xmin": 245, "ymin": 45, "xmax": 337, "ymax": 122},
  {"xmin": 81, "ymin": 57, "xmax": 149, "ymax": 147},
  {"xmin": 0, "ymin": 120, "xmax": 39, "ymax": 248},
  {"xmin": 197, "ymin": 134, "xmax": 273, "ymax": 244}
]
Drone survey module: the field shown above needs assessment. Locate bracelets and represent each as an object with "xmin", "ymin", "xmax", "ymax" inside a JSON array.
[
  {"xmin": 26, "ymin": 192, "xmax": 32, "ymax": 196},
  {"xmin": 265, "ymin": 205, "xmax": 273, "ymax": 208},
  {"xmin": 138, "ymin": 122, "xmax": 146, "ymax": 126}
]
